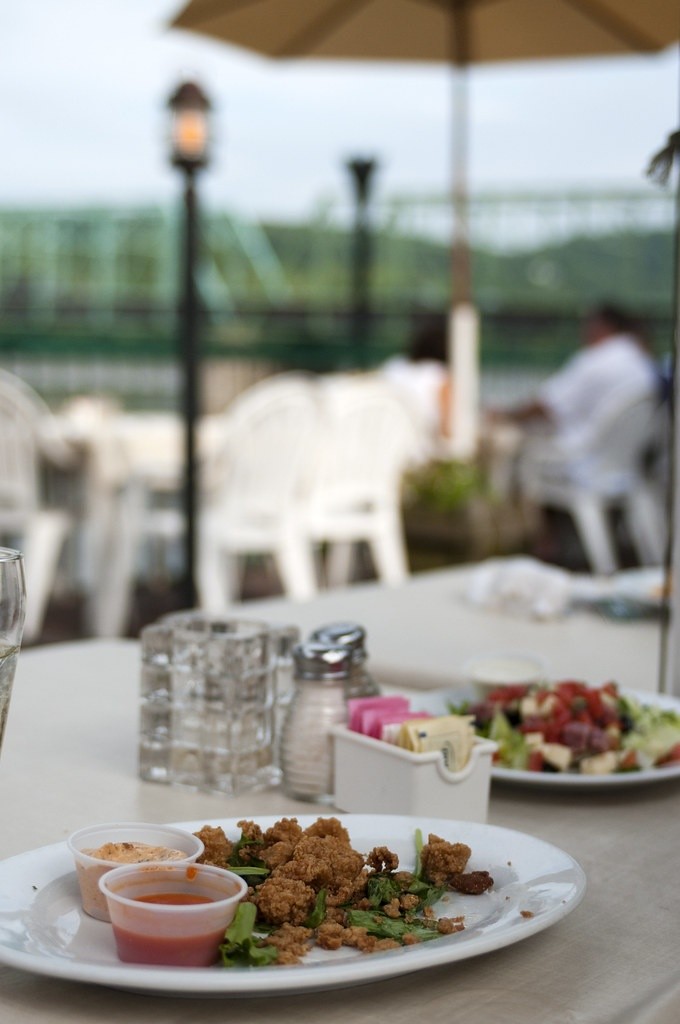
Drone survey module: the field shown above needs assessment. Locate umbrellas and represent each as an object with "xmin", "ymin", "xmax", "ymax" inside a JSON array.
[{"xmin": 172, "ymin": 0, "xmax": 680, "ymax": 305}]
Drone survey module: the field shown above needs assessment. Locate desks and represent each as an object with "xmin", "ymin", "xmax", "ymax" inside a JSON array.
[{"xmin": 0, "ymin": 557, "xmax": 680, "ymax": 1024}]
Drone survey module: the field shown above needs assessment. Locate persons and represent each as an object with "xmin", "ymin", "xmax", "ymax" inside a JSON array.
[{"xmin": 499, "ymin": 300, "xmax": 658, "ymax": 573}]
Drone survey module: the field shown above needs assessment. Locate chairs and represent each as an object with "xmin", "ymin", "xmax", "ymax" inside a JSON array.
[{"xmin": 0, "ymin": 357, "xmax": 680, "ymax": 649}]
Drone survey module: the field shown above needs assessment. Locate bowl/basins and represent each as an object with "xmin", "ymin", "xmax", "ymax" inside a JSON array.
[
  {"xmin": 67, "ymin": 822, "xmax": 205, "ymax": 922},
  {"xmin": 100, "ymin": 860, "xmax": 247, "ymax": 967}
]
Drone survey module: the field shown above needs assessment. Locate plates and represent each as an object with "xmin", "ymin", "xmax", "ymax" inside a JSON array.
[
  {"xmin": 417, "ymin": 683, "xmax": 680, "ymax": 785},
  {"xmin": 1, "ymin": 815, "xmax": 587, "ymax": 1000}
]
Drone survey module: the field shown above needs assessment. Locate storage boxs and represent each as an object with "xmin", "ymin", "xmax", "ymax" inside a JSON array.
[{"xmin": 332, "ymin": 723, "xmax": 499, "ymax": 827}]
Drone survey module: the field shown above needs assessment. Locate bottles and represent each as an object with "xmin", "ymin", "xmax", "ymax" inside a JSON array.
[{"xmin": 279, "ymin": 619, "xmax": 378, "ymax": 802}]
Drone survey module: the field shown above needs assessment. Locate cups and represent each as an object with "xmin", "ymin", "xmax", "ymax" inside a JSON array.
[
  {"xmin": 0, "ymin": 546, "xmax": 27, "ymax": 746},
  {"xmin": 141, "ymin": 611, "xmax": 303, "ymax": 793}
]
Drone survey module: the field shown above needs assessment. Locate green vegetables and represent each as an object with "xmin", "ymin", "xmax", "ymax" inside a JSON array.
[
  {"xmin": 218, "ymin": 829, "xmax": 449, "ymax": 969},
  {"xmin": 454, "ymin": 686, "xmax": 676, "ymax": 772}
]
